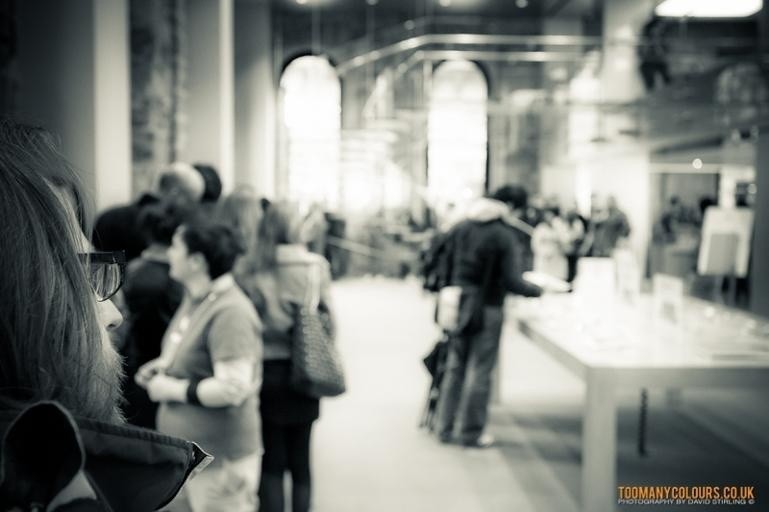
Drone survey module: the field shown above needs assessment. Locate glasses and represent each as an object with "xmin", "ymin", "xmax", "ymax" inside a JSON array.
[{"xmin": 74, "ymin": 248, "xmax": 125, "ymax": 301}]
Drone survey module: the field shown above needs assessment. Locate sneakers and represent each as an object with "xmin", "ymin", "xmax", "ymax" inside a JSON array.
[
  {"xmin": 458, "ymin": 432, "xmax": 496, "ymax": 448},
  {"xmin": 434, "ymin": 431, "xmax": 462, "ymax": 443}
]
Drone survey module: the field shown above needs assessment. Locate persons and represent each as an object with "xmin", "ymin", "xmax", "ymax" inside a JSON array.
[
  {"xmin": 88, "ymin": 162, "xmax": 347, "ymax": 511},
  {"xmin": 0, "ymin": 120, "xmax": 213, "ymax": 511},
  {"xmin": 363, "ymin": 175, "xmax": 757, "ymax": 307},
  {"xmin": 422, "ymin": 184, "xmax": 545, "ymax": 450}
]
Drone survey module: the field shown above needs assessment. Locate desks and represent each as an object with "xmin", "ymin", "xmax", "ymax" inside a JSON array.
[{"xmin": 491, "ymin": 293, "xmax": 769, "ymax": 512}]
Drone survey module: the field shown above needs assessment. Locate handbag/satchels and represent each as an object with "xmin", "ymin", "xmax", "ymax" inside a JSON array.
[
  {"xmin": 286, "ymin": 300, "xmax": 347, "ymax": 397},
  {"xmin": 435, "ymin": 285, "xmax": 487, "ymax": 337}
]
